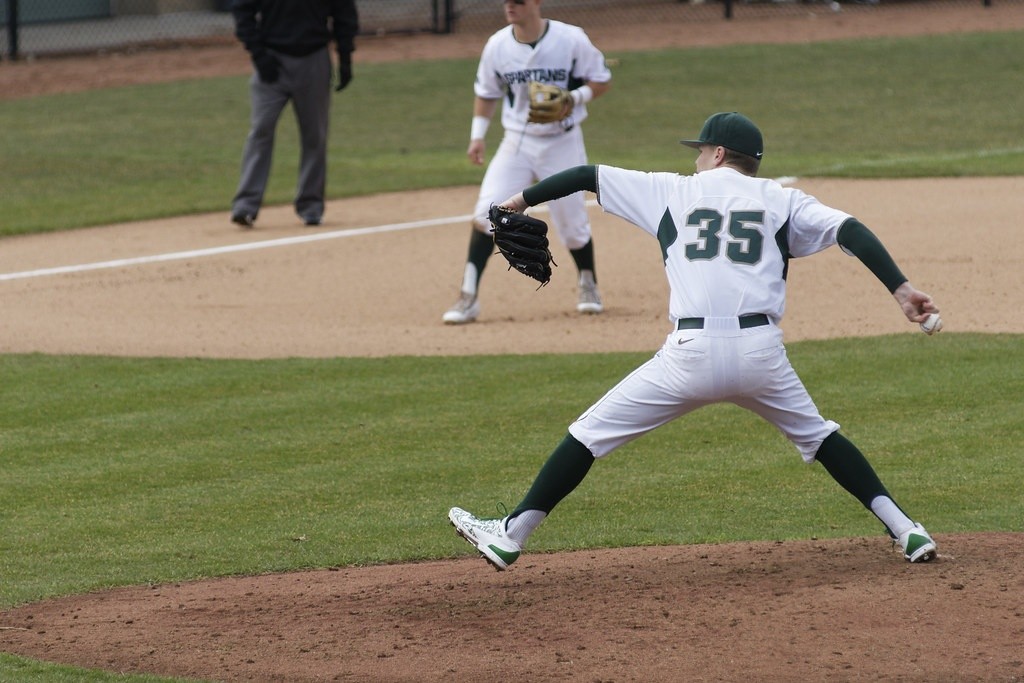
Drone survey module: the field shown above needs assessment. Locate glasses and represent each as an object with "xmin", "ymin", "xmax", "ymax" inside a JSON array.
[{"xmin": 503, "ymin": 0, "xmax": 526, "ymax": 5}]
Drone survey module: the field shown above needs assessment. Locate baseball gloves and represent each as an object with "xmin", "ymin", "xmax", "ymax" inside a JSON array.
[
  {"xmin": 528, "ymin": 81, "xmax": 574, "ymax": 124},
  {"xmin": 488, "ymin": 205, "xmax": 552, "ymax": 283}
]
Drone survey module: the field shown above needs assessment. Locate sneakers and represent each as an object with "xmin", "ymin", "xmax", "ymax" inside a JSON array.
[
  {"xmin": 576, "ymin": 276, "xmax": 603, "ymax": 313},
  {"xmin": 447, "ymin": 506, "xmax": 522, "ymax": 574},
  {"xmin": 441, "ymin": 291, "xmax": 482, "ymax": 326},
  {"xmin": 302, "ymin": 210, "xmax": 321, "ymax": 225},
  {"xmin": 893, "ymin": 522, "xmax": 938, "ymax": 564},
  {"xmin": 231, "ymin": 207, "xmax": 253, "ymax": 227}
]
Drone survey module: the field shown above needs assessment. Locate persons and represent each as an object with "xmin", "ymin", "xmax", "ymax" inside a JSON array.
[
  {"xmin": 230, "ymin": 0, "xmax": 359, "ymax": 226},
  {"xmin": 441, "ymin": 0, "xmax": 612, "ymax": 325},
  {"xmin": 448, "ymin": 112, "xmax": 943, "ymax": 573}
]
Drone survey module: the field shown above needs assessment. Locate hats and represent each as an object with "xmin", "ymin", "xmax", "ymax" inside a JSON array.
[{"xmin": 680, "ymin": 111, "xmax": 763, "ymax": 162}]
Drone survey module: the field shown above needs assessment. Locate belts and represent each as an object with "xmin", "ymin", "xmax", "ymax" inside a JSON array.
[
  {"xmin": 566, "ymin": 125, "xmax": 573, "ymax": 133},
  {"xmin": 677, "ymin": 314, "xmax": 769, "ymax": 330}
]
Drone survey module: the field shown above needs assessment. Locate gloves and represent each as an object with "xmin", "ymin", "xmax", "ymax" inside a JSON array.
[
  {"xmin": 336, "ymin": 51, "xmax": 354, "ymax": 92},
  {"xmin": 249, "ymin": 43, "xmax": 285, "ymax": 85}
]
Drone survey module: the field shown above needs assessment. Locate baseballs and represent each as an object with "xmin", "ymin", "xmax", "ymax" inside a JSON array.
[{"xmin": 920, "ymin": 313, "xmax": 942, "ymax": 334}]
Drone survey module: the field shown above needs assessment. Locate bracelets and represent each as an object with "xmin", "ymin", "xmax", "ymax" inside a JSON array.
[{"xmin": 471, "ymin": 116, "xmax": 491, "ymax": 140}]
